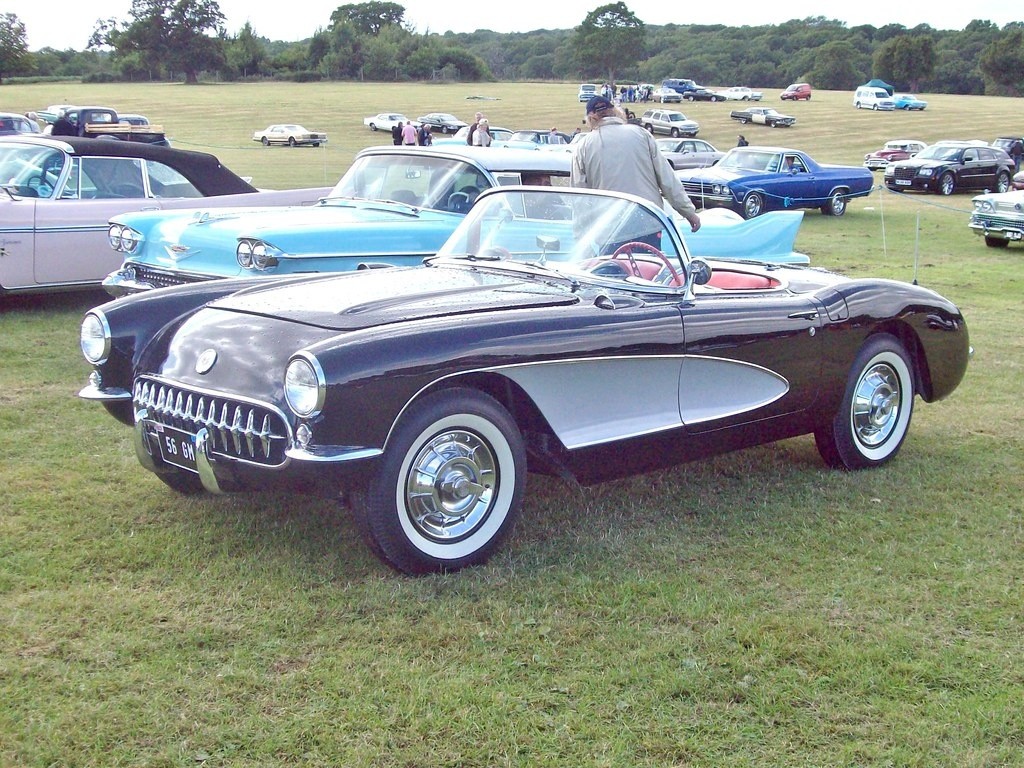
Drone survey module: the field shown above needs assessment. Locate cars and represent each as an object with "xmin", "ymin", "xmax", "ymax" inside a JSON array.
[
  {"xmin": 534, "ymin": 133, "xmax": 588, "ymax": 153},
  {"xmin": 364, "ymin": 114, "xmax": 468, "ymax": 134},
  {"xmin": 252, "ymin": 124, "xmax": 328, "ymax": 147},
  {"xmin": 658, "ymin": 146, "xmax": 874, "ymax": 221},
  {"xmin": 884, "ymin": 141, "xmax": 1016, "ymax": 196},
  {"xmin": 0, "ymin": 112, "xmax": 41, "ymax": 136},
  {"xmin": 0, "ymin": 134, "xmax": 336, "ymax": 294},
  {"xmin": 100, "ymin": 146, "xmax": 811, "ymax": 300},
  {"xmin": 730, "ymin": 106, "xmax": 797, "ymax": 128},
  {"xmin": 982, "ymin": 136, "xmax": 1024, "ymax": 159},
  {"xmin": 655, "ymin": 138, "xmax": 727, "ymax": 171},
  {"xmin": 490, "ymin": 130, "xmax": 572, "ymax": 150},
  {"xmin": 431, "ymin": 126, "xmax": 514, "ymax": 146},
  {"xmin": 578, "ymin": 79, "xmax": 811, "ymax": 103},
  {"xmin": 640, "ymin": 109, "xmax": 700, "ymax": 138},
  {"xmin": 968, "ymin": 171, "xmax": 1024, "ymax": 247},
  {"xmin": 79, "ymin": 185, "xmax": 975, "ymax": 576},
  {"xmin": 863, "ymin": 140, "xmax": 929, "ymax": 171},
  {"xmin": 853, "ymin": 87, "xmax": 928, "ymax": 110},
  {"xmin": 35, "ymin": 105, "xmax": 171, "ymax": 164}
]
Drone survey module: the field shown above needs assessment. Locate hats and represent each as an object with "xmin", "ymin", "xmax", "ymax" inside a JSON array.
[
  {"xmin": 587, "ymin": 96, "xmax": 614, "ymax": 114},
  {"xmin": 479, "ymin": 118, "xmax": 489, "ymax": 125}
]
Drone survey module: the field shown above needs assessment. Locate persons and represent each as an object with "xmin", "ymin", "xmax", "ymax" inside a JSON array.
[
  {"xmin": 570, "ymin": 128, "xmax": 580, "ymax": 142},
  {"xmin": 22, "ymin": 112, "xmax": 78, "ymax": 137},
  {"xmin": 467, "ymin": 114, "xmax": 492, "ymax": 147},
  {"xmin": 391, "ymin": 121, "xmax": 433, "ymax": 147},
  {"xmin": 784, "ymin": 156, "xmax": 800, "ymax": 172},
  {"xmin": 519, "ymin": 171, "xmax": 564, "ymax": 219},
  {"xmin": 548, "ymin": 128, "xmax": 561, "ymax": 144},
  {"xmin": 737, "ymin": 136, "xmax": 748, "ymax": 147},
  {"xmin": 1012, "ymin": 137, "xmax": 1023, "ymax": 171},
  {"xmin": 569, "ymin": 95, "xmax": 701, "ymax": 256},
  {"xmin": 600, "ymin": 82, "xmax": 651, "ymax": 102}
]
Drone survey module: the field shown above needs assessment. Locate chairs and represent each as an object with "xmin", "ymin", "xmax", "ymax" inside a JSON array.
[{"xmin": 392, "ymin": 190, "xmax": 417, "ymax": 206}]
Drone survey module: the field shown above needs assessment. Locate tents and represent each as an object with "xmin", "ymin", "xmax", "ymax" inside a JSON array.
[{"xmin": 862, "ymin": 78, "xmax": 895, "ymax": 96}]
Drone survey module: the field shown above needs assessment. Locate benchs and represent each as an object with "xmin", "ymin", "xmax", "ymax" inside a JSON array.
[{"xmin": 620, "ymin": 257, "xmax": 779, "ymax": 289}]
